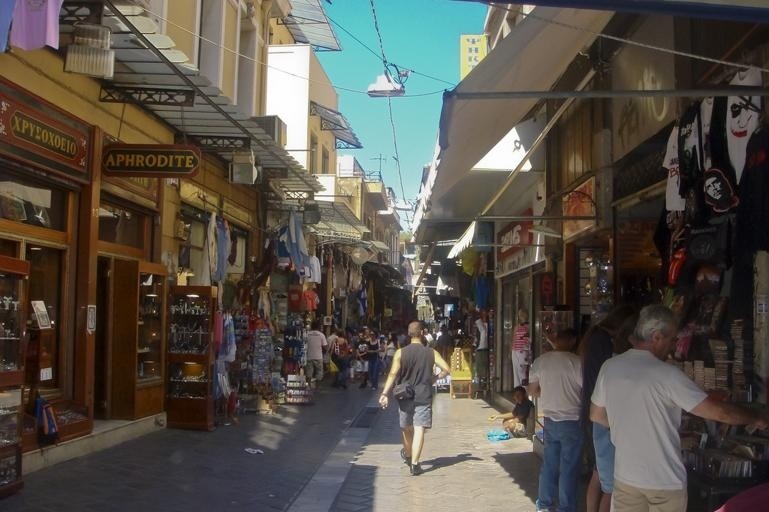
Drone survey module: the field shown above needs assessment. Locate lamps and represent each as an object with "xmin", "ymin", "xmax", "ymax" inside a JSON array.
[
  {"xmin": 302, "ymin": 198, "xmax": 321, "ymax": 224},
  {"xmin": 63, "ymin": 21, "xmax": 116, "ymax": 79},
  {"xmin": 226, "ymin": 148, "xmax": 258, "ymax": 186}
]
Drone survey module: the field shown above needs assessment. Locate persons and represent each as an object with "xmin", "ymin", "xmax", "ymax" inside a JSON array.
[
  {"xmin": 473, "ymin": 315, "xmax": 489, "ymax": 382},
  {"xmin": 581, "ymin": 301, "xmax": 637, "ymax": 511},
  {"xmin": 306, "ymin": 318, "xmax": 456, "ymax": 398},
  {"xmin": 529, "ymin": 326, "xmax": 585, "ymax": 511},
  {"xmin": 511, "ymin": 307, "xmax": 530, "ymax": 396},
  {"xmin": 377, "ymin": 320, "xmax": 451, "ymax": 475},
  {"xmin": 589, "ymin": 303, "xmax": 769, "ymax": 512},
  {"xmin": 701, "ymin": 173, "xmax": 727, "ymax": 201},
  {"xmin": 486, "ymin": 385, "xmax": 535, "ymax": 438}
]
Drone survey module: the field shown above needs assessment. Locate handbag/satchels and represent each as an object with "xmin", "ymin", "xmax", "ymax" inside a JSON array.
[{"xmin": 392, "ymin": 383, "xmax": 414, "ymax": 400}]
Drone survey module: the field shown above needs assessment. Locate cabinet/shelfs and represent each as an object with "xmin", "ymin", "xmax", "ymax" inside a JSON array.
[
  {"xmin": 111, "ymin": 260, "xmax": 168, "ymax": 421},
  {"xmin": 0, "ymin": 255, "xmax": 30, "ymax": 392},
  {"xmin": 166, "ymin": 286, "xmax": 222, "ymax": 432}
]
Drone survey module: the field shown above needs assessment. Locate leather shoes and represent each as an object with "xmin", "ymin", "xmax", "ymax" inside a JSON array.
[{"xmin": 400, "ymin": 447, "xmax": 424, "ymax": 475}]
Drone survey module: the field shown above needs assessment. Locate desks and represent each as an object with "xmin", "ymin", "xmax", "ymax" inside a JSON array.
[{"xmin": 450, "ymin": 379, "xmax": 472, "ymax": 398}]
